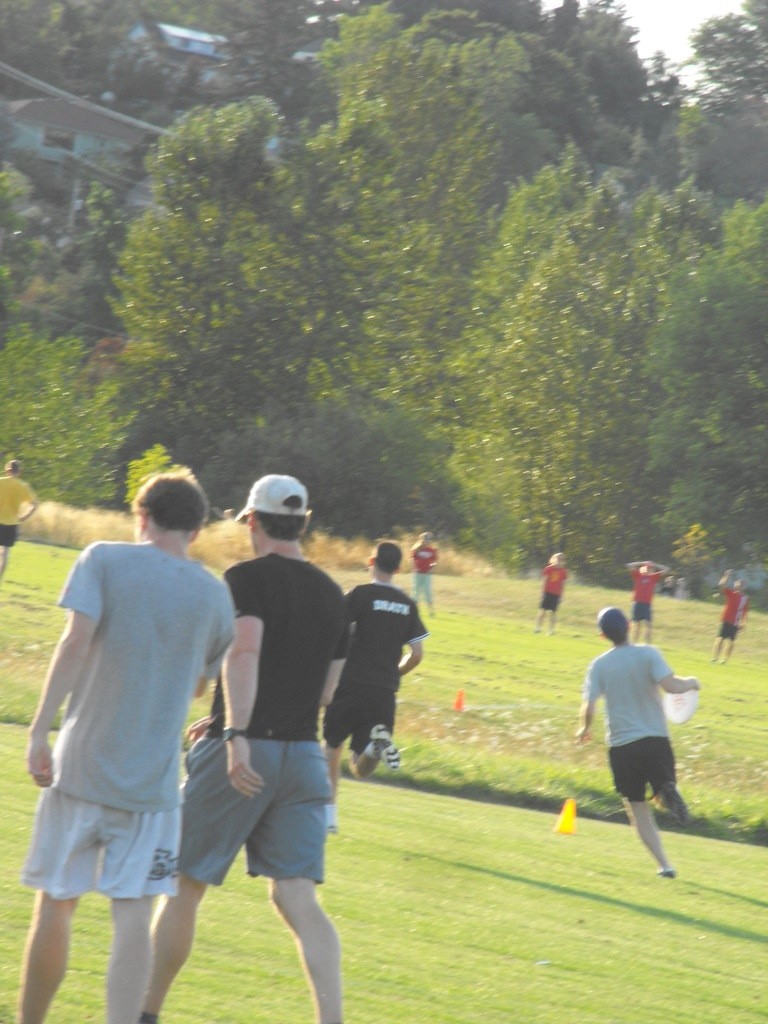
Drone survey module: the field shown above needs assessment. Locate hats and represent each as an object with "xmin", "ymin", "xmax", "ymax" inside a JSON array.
[
  {"xmin": 597, "ymin": 607, "xmax": 626, "ymax": 638},
  {"xmin": 234, "ymin": 474, "xmax": 308, "ymax": 522}
]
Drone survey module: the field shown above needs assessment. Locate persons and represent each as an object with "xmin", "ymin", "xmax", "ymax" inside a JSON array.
[
  {"xmin": 137, "ymin": 473, "xmax": 351, "ymax": 1024},
  {"xmin": 0, "ymin": 460, "xmax": 35, "ymax": 578},
  {"xmin": 577, "ymin": 607, "xmax": 700, "ymax": 878},
  {"xmin": 326, "ymin": 542, "xmax": 429, "ymax": 831},
  {"xmin": 369, "ymin": 532, "xmax": 751, "ymax": 667},
  {"xmin": 15, "ymin": 472, "xmax": 233, "ymax": 1023}
]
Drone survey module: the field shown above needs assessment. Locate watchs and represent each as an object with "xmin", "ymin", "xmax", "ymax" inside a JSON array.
[{"xmin": 222, "ymin": 728, "xmax": 245, "ymax": 741}]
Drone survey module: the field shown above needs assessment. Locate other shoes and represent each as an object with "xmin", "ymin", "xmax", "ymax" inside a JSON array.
[
  {"xmin": 370, "ymin": 724, "xmax": 401, "ymax": 769},
  {"xmin": 328, "ymin": 822, "xmax": 337, "ymax": 831},
  {"xmin": 656, "ymin": 869, "xmax": 677, "ymax": 879},
  {"xmin": 664, "ymin": 781, "xmax": 688, "ymax": 824}
]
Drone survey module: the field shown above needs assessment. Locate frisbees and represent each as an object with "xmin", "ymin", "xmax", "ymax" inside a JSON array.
[{"xmin": 661, "ymin": 681, "xmax": 698, "ymax": 725}]
